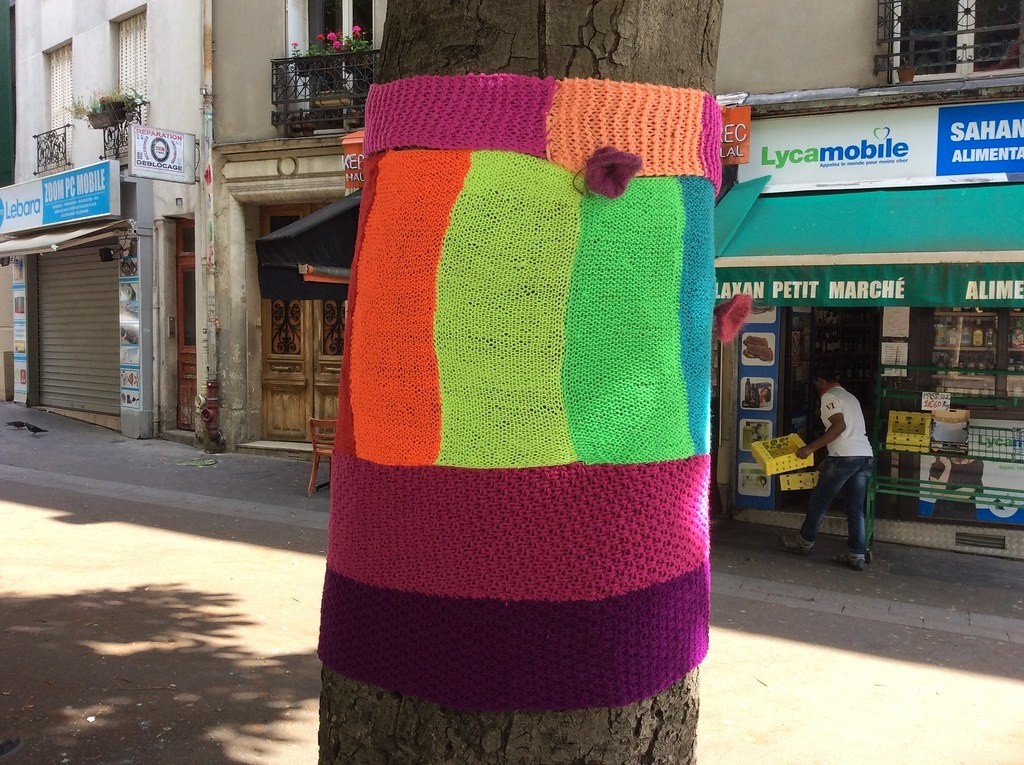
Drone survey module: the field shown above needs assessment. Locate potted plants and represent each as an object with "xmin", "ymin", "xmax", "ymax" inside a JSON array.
[
  {"xmin": 60, "ymin": 89, "xmax": 118, "ymax": 130},
  {"xmin": 896, "ymin": 56, "xmax": 917, "ymax": 83}
]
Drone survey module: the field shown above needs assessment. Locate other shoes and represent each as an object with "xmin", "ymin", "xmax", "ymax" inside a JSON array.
[
  {"xmin": 847, "ymin": 551, "xmax": 865, "ymax": 570},
  {"xmin": 779, "ymin": 533, "xmax": 815, "ymax": 555}
]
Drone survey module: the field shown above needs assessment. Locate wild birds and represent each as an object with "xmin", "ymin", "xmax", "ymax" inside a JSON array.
[{"xmin": 5, "ymin": 421, "xmax": 49, "ymax": 437}]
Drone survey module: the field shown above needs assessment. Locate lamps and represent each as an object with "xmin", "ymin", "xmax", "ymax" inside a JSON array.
[
  {"xmin": 100, "ymin": 248, "xmax": 120, "ymax": 262},
  {"xmin": 0, "ymin": 256, "xmax": 18, "ymax": 267}
]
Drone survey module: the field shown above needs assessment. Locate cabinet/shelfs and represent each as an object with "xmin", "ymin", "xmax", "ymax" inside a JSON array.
[
  {"xmin": 806, "ymin": 307, "xmax": 881, "ymax": 444},
  {"xmin": 932, "ymin": 310, "xmax": 1024, "ymax": 382}
]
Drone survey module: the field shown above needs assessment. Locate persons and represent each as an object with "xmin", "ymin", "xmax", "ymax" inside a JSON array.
[{"xmin": 776, "ymin": 364, "xmax": 875, "ymax": 570}]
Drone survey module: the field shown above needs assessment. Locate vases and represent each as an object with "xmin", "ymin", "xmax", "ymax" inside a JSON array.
[
  {"xmin": 297, "ymin": 53, "xmax": 370, "ymax": 77},
  {"xmin": 315, "ymin": 90, "xmax": 351, "ymax": 108},
  {"xmin": 106, "ymin": 99, "xmax": 134, "ymax": 125}
]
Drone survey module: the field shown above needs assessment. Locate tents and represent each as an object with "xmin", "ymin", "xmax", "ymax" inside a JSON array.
[{"xmin": 254, "ymin": 188, "xmax": 362, "ymax": 300}]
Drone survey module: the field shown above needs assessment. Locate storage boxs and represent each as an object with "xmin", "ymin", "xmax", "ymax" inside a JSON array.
[
  {"xmin": 886, "ymin": 410, "xmax": 932, "ymax": 453},
  {"xmin": 779, "ymin": 466, "xmax": 819, "ymax": 490},
  {"xmin": 751, "ymin": 432, "xmax": 814, "ymax": 477},
  {"xmin": 930, "ymin": 408, "xmax": 970, "ymax": 455}
]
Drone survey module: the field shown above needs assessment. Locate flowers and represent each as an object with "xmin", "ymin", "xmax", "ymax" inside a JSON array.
[
  {"xmin": 291, "ymin": 25, "xmax": 373, "ymax": 58},
  {"xmin": 99, "ymin": 87, "xmax": 134, "ymax": 113}
]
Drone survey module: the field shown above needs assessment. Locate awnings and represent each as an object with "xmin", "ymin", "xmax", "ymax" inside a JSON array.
[
  {"xmin": 0, "ymin": 219, "xmax": 137, "ymax": 259},
  {"xmin": 712, "ymin": 175, "xmax": 1024, "ymax": 308}
]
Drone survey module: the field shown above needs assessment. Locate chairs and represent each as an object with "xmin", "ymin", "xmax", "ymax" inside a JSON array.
[{"xmin": 306, "ymin": 416, "xmax": 338, "ymax": 500}]
